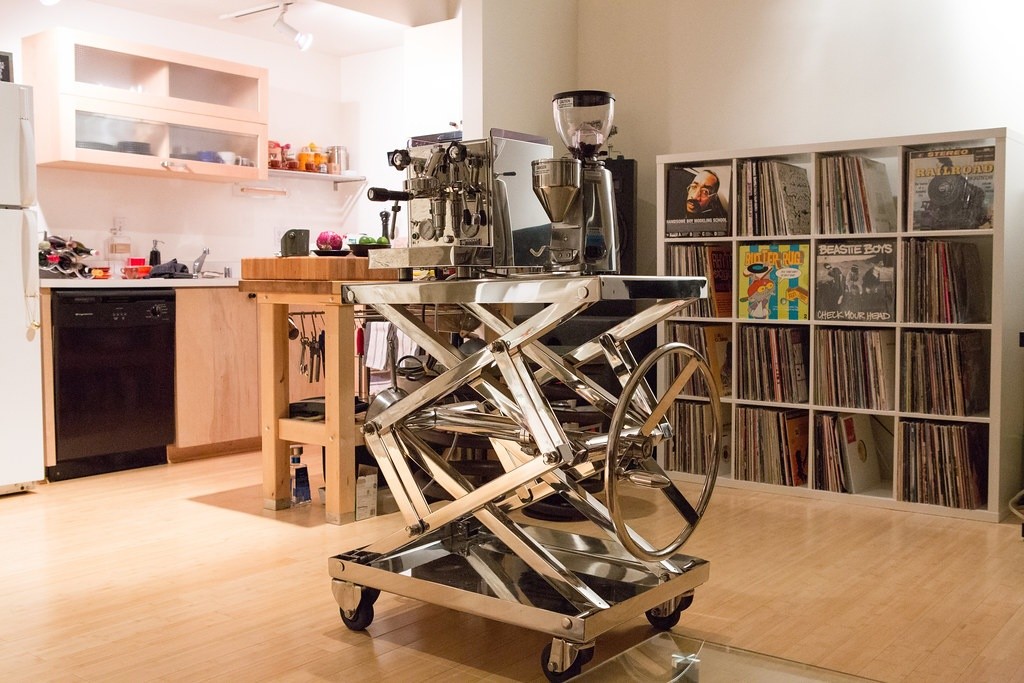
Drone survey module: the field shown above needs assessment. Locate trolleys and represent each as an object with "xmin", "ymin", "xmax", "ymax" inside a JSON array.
[{"xmin": 326, "ymin": 270, "xmax": 725, "ymax": 683}]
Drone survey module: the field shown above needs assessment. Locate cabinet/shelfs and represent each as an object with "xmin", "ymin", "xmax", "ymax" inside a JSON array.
[
  {"xmin": 168, "ymin": 289, "xmax": 364, "ymax": 470},
  {"xmin": 655, "ymin": 127, "xmax": 1024, "ymax": 522},
  {"xmin": 22, "ymin": 27, "xmax": 270, "ymax": 181}
]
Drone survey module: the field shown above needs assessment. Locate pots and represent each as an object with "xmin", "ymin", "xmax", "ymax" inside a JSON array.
[{"xmin": 363, "ymin": 343, "xmax": 408, "ymax": 423}]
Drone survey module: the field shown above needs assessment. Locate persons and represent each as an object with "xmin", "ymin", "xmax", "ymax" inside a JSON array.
[{"xmin": 672, "ymin": 169, "xmax": 723, "ymax": 219}]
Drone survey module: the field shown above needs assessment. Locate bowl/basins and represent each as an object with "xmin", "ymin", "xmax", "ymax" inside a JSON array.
[
  {"xmin": 130, "ymin": 266, "xmax": 151, "ymax": 278},
  {"xmin": 94, "ymin": 267, "xmax": 109, "ymax": 273},
  {"xmin": 348, "ymin": 244, "xmax": 392, "ymax": 257}
]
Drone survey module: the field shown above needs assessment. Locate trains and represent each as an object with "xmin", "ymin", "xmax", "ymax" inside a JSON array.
[{"xmin": 919, "ymin": 164, "xmax": 985, "ymax": 231}]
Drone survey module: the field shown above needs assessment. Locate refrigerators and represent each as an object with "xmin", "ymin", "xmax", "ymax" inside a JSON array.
[{"xmin": 0, "ymin": 78, "xmax": 45, "ymax": 497}]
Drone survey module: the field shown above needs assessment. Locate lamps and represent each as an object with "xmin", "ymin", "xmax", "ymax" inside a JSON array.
[{"xmin": 273, "ymin": 5, "xmax": 313, "ymax": 52}]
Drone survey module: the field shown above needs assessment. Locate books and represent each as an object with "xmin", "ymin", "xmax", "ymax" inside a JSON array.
[{"xmin": 661, "ymin": 146, "xmax": 996, "ymax": 510}]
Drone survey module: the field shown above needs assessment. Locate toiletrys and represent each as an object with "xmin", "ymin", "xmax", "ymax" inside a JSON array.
[{"xmin": 149, "ymin": 240, "xmax": 165, "ymax": 268}]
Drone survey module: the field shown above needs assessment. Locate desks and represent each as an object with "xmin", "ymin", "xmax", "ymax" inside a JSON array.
[{"xmin": 239, "ymin": 277, "xmax": 428, "ymax": 526}]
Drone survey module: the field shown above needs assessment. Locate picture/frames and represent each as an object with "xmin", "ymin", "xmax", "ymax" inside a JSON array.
[{"xmin": 0, "ymin": 51, "xmax": 14, "ymax": 84}]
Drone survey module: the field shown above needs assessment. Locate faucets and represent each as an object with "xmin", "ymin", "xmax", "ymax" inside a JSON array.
[{"xmin": 193, "ymin": 247, "xmax": 210, "ymax": 280}]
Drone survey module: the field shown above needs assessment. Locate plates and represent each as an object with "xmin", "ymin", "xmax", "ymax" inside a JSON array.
[
  {"xmin": 78, "ymin": 141, "xmax": 153, "ymax": 156},
  {"xmin": 170, "ymin": 154, "xmax": 201, "ymax": 162},
  {"xmin": 311, "ymin": 249, "xmax": 352, "ymax": 256}
]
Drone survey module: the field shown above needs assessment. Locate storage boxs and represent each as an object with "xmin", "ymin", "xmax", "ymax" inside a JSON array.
[
  {"xmin": 377, "ymin": 485, "xmax": 400, "ymax": 513},
  {"xmin": 356, "ymin": 463, "xmax": 379, "ymax": 522}
]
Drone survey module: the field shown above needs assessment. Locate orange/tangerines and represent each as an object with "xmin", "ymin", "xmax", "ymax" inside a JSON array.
[
  {"xmin": 377, "ymin": 236, "xmax": 388, "ymax": 243},
  {"xmin": 359, "ymin": 236, "xmax": 369, "ymax": 243},
  {"xmin": 369, "ymin": 237, "xmax": 375, "ymax": 243}
]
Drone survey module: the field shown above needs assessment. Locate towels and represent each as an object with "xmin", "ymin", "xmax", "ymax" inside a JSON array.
[{"xmin": 363, "ymin": 322, "xmax": 420, "ymax": 396}]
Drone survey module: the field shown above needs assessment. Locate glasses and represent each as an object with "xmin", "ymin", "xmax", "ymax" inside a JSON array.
[{"xmin": 686, "ymin": 185, "xmax": 716, "ymax": 198}]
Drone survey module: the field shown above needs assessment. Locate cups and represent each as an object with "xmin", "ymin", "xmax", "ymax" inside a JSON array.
[
  {"xmin": 327, "ymin": 163, "xmax": 339, "ymax": 174},
  {"xmin": 286, "ymin": 162, "xmax": 298, "ymax": 168},
  {"xmin": 121, "ymin": 268, "xmax": 138, "ymax": 279},
  {"xmin": 305, "ymin": 164, "xmax": 313, "ymax": 170},
  {"xmin": 198, "ymin": 151, "xmax": 224, "ymax": 164},
  {"xmin": 268, "ymin": 160, "xmax": 279, "ymax": 167},
  {"xmin": 218, "ymin": 151, "xmax": 242, "ymax": 166}
]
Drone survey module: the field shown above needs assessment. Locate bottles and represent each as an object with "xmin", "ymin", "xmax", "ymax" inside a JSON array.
[
  {"xmin": 290, "ymin": 456, "xmax": 312, "ymax": 505},
  {"xmin": 150, "ymin": 240, "xmax": 161, "ymax": 266},
  {"xmin": 39, "ymin": 236, "xmax": 103, "ymax": 277}
]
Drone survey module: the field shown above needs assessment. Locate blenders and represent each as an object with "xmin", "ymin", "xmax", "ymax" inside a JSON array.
[{"xmin": 526, "ymin": 89, "xmax": 639, "ymax": 276}]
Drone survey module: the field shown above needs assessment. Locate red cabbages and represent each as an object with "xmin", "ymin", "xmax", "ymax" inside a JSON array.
[{"xmin": 316, "ymin": 231, "xmax": 342, "ymax": 250}]
